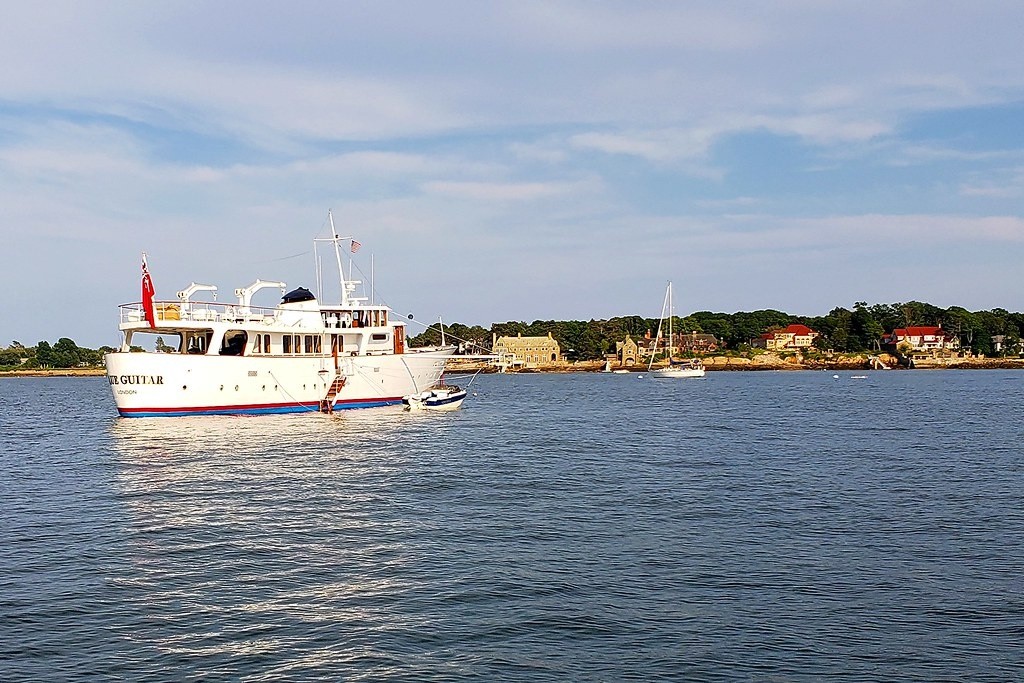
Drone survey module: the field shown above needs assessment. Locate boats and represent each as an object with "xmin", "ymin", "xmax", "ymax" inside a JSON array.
[
  {"xmin": 399, "ymin": 356, "xmax": 468, "ymax": 413},
  {"xmin": 103, "ymin": 207, "xmax": 504, "ymax": 418}
]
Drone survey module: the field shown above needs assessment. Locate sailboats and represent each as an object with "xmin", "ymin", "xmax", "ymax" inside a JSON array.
[{"xmin": 646, "ymin": 280, "xmax": 707, "ymax": 378}]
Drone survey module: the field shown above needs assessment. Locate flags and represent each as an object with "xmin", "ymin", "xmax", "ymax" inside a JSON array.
[
  {"xmin": 351, "ymin": 239, "xmax": 362, "ymax": 253},
  {"xmin": 141, "ymin": 258, "xmax": 156, "ymax": 329}
]
